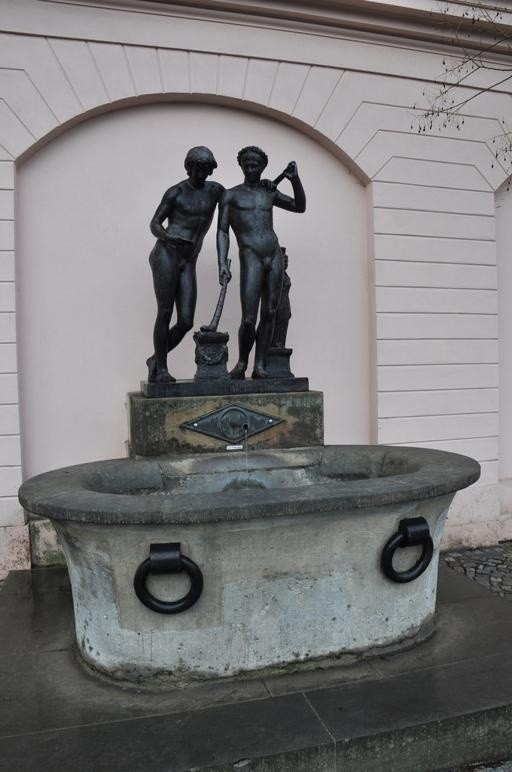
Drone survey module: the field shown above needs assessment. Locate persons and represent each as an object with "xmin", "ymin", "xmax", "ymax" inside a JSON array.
[
  {"xmin": 146, "ymin": 146, "xmax": 226, "ymax": 383},
  {"xmin": 260, "ymin": 247, "xmax": 292, "ymax": 347},
  {"xmin": 216, "ymin": 146, "xmax": 305, "ymax": 379}
]
